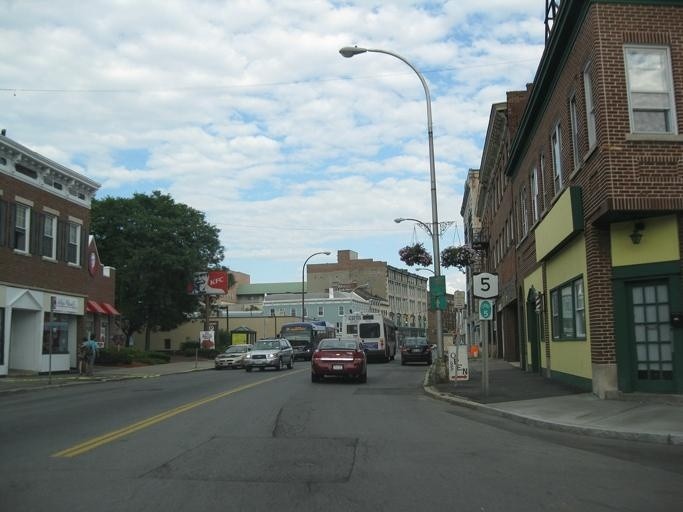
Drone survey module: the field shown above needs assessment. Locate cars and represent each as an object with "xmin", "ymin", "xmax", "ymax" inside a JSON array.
[
  {"xmin": 401, "ymin": 336, "xmax": 433, "ymax": 366},
  {"xmin": 214, "ymin": 339, "xmax": 367, "ymax": 383}
]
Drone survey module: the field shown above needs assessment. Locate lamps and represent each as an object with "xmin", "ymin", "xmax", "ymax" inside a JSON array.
[{"xmin": 630, "ymin": 227, "xmax": 642, "ymax": 244}]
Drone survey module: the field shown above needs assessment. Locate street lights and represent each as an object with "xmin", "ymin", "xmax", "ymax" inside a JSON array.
[
  {"xmin": 339, "ymin": 46, "xmax": 443, "ymax": 364},
  {"xmin": 394, "ymin": 218, "xmax": 433, "ymax": 237},
  {"xmin": 415, "ymin": 268, "xmax": 435, "ymax": 275},
  {"xmin": 302, "ymin": 251, "xmax": 331, "ymax": 322}
]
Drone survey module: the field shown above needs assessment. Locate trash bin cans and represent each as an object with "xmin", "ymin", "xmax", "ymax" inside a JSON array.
[{"xmin": 471, "ymin": 345, "xmax": 478, "ymax": 357}]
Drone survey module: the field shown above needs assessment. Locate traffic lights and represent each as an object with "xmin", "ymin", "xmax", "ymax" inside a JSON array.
[{"xmin": 51, "ymin": 296, "xmax": 56, "ymax": 310}]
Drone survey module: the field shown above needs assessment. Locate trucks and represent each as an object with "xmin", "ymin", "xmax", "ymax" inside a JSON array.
[{"xmin": 277, "ymin": 312, "xmax": 396, "ymax": 361}]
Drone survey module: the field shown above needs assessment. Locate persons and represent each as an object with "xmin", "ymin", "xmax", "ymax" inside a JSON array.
[
  {"xmin": 76, "ymin": 336, "xmax": 88, "ymax": 374},
  {"xmin": 85, "ymin": 333, "xmax": 98, "ymax": 377}
]
